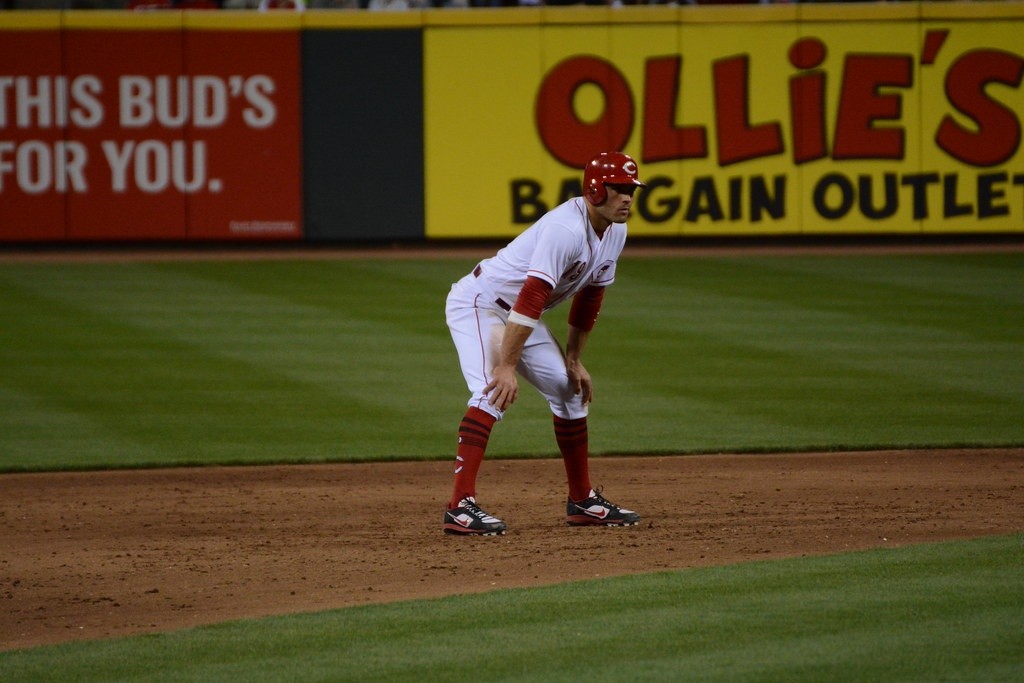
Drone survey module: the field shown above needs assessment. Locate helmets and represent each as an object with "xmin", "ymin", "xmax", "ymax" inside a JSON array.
[{"xmin": 583, "ymin": 152, "xmax": 647, "ymax": 205}]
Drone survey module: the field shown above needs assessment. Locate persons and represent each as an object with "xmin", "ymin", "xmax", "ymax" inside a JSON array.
[{"xmin": 443, "ymin": 151, "xmax": 645, "ymax": 537}]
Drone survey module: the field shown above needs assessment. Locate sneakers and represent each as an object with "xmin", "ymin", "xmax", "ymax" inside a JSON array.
[
  {"xmin": 443, "ymin": 492, "xmax": 506, "ymax": 536},
  {"xmin": 566, "ymin": 484, "xmax": 640, "ymax": 526}
]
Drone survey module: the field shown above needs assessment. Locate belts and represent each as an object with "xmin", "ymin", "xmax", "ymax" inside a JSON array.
[{"xmin": 473, "ymin": 265, "xmax": 511, "ymax": 312}]
